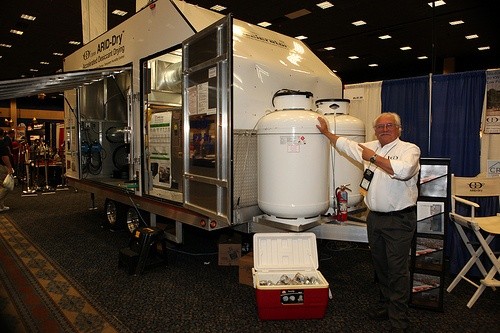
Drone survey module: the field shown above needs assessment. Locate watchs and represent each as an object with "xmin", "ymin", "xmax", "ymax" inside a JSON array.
[{"xmin": 370, "ymin": 154, "xmax": 377, "ymax": 163}]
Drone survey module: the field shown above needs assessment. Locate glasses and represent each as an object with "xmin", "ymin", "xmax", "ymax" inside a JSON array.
[{"xmin": 376, "ymin": 123, "xmax": 397, "ymax": 129}]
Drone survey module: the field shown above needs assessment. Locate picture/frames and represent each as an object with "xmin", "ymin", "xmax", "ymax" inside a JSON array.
[
  {"xmin": 416, "ymin": 158, "xmax": 450, "ymax": 200},
  {"xmin": 415, "ymin": 200, "xmax": 446, "ymax": 240}
]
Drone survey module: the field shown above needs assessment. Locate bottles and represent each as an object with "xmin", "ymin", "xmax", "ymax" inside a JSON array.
[{"xmin": 259, "ymin": 272, "xmax": 321, "ymax": 285}]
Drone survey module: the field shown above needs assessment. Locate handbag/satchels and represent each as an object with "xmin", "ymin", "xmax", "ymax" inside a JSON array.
[{"xmin": 3, "ymin": 173, "xmax": 14, "ymax": 190}]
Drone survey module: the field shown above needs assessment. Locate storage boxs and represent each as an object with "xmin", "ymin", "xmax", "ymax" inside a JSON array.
[
  {"xmin": 218, "ymin": 244, "xmax": 241, "ymax": 266},
  {"xmin": 251, "ymin": 232, "xmax": 334, "ymax": 320},
  {"xmin": 239, "ymin": 251, "xmax": 253, "ymax": 286}
]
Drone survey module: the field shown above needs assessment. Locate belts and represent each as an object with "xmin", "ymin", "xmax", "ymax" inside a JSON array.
[{"xmin": 372, "ymin": 206, "xmax": 415, "ymax": 216}]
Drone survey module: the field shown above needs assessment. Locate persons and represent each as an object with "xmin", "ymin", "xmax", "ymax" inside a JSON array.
[
  {"xmin": 0, "ymin": 129, "xmax": 27, "ymax": 213},
  {"xmin": 316, "ymin": 111, "xmax": 421, "ymax": 333}
]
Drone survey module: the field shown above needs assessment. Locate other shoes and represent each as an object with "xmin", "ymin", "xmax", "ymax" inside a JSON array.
[
  {"xmin": 0, "ymin": 206, "xmax": 9, "ymax": 211},
  {"xmin": 369, "ymin": 307, "xmax": 388, "ymax": 321},
  {"xmin": 389, "ymin": 320, "xmax": 406, "ymax": 333}
]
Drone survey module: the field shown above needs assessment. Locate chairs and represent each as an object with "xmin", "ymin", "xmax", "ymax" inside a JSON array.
[{"xmin": 447, "ymin": 175, "xmax": 500, "ymax": 308}]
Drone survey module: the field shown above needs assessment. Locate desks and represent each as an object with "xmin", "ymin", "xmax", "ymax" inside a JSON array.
[{"xmin": 34, "ymin": 162, "xmax": 66, "ymax": 185}]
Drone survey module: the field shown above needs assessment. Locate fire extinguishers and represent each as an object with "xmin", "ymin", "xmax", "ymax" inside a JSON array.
[{"xmin": 335, "ymin": 184, "xmax": 352, "ymax": 222}]
